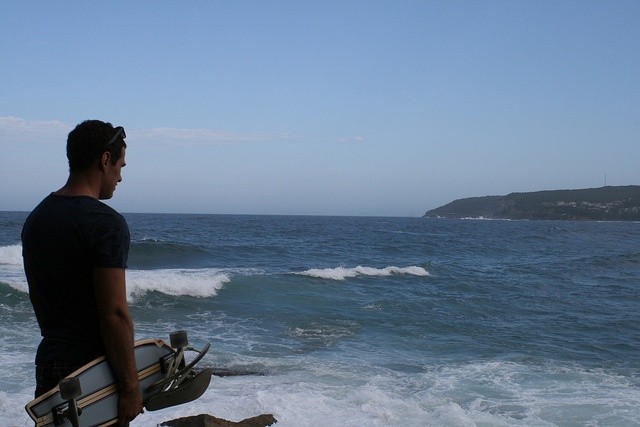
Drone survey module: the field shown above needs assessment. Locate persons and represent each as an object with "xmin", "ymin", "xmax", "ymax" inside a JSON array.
[{"xmin": 22, "ymin": 120, "xmax": 144, "ymax": 427}]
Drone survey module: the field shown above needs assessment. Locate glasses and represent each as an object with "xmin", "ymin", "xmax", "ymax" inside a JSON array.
[{"xmin": 104, "ymin": 124, "xmax": 126, "ymax": 151}]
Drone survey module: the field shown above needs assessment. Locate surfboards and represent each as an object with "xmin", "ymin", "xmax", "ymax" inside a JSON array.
[{"xmin": 25, "ymin": 325, "xmax": 209, "ymax": 427}]
